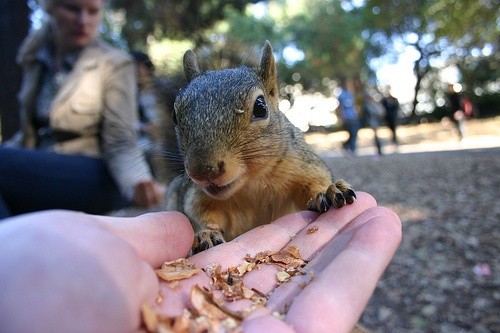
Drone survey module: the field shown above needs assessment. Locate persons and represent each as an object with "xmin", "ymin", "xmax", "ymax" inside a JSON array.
[
  {"xmin": 0, "ymin": 0, "xmax": 167, "ymax": 222},
  {"xmin": 338, "ymin": 78, "xmax": 463, "ymax": 158},
  {"xmin": 130, "ymin": 51, "xmax": 169, "ymax": 184},
  {"xmin": 1, "ymin": 189, "xmax": 403, "ymax": 333}
]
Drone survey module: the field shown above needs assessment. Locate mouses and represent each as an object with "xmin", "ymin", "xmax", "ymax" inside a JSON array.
[{"xmin": 154, "ymin": 40, "xmax": 357, "ymax": 259}]
